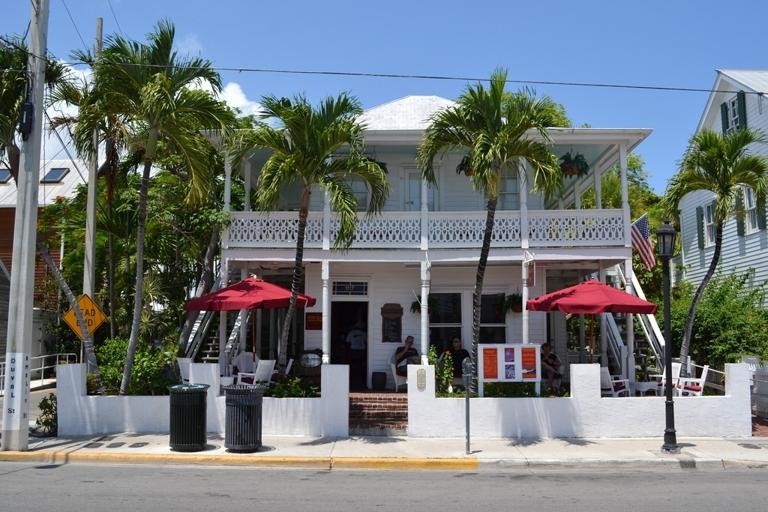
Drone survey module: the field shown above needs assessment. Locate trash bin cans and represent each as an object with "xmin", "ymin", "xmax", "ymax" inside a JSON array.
[
  {"xmin": 222, "ymin": 384, "xmax": 270, "ymax": 453},
  {"xmin": 166, "ymin": 384, "xmax": 210, "ymax": 452}
]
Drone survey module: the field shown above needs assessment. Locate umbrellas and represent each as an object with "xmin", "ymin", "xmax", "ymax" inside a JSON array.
[
  {"xmin": 181, "ymin": 274, "xmax": 317, "ymax": 373},
  {"xmin": 524, "ymin": 275, "xmax": 659, "ymax": 363}
]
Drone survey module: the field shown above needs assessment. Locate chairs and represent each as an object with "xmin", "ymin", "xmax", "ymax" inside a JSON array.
[
  {"xmin": 599, "ymin": 360, "xmax": 710, "ymax": 397},
  {"xmin": 387, "ymin": 353, "xmax": 407, "ymax": 393},
  {"xmin": 176, "ymin": 350, "xmax": 294, "ymax": 396}
]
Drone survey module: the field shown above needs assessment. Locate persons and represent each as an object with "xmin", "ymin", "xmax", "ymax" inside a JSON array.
[
  {"xmin": 539, "ymin": 340, "xmax": 564, "ymax": 397},
  {"xmin": 440, "ymin": 336, "xmax": 472, "ymax": 394},
  {"xmin": 345, "ymin": 318, "xmax": 367, "ymax": 390},
  {"xmin": 395, "ymin": 336, "xmax": 421, "ymax": 376}
]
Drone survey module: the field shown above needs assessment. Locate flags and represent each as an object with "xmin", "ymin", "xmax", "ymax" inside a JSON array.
[{"xmin": 630, "ymin": 213, "xmax": 658, "ymax": 273}]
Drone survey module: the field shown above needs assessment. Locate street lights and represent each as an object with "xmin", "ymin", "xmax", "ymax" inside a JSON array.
[{"xmin": 654, "ymin": 217, "xmax": 687, "ymax": 451}]
